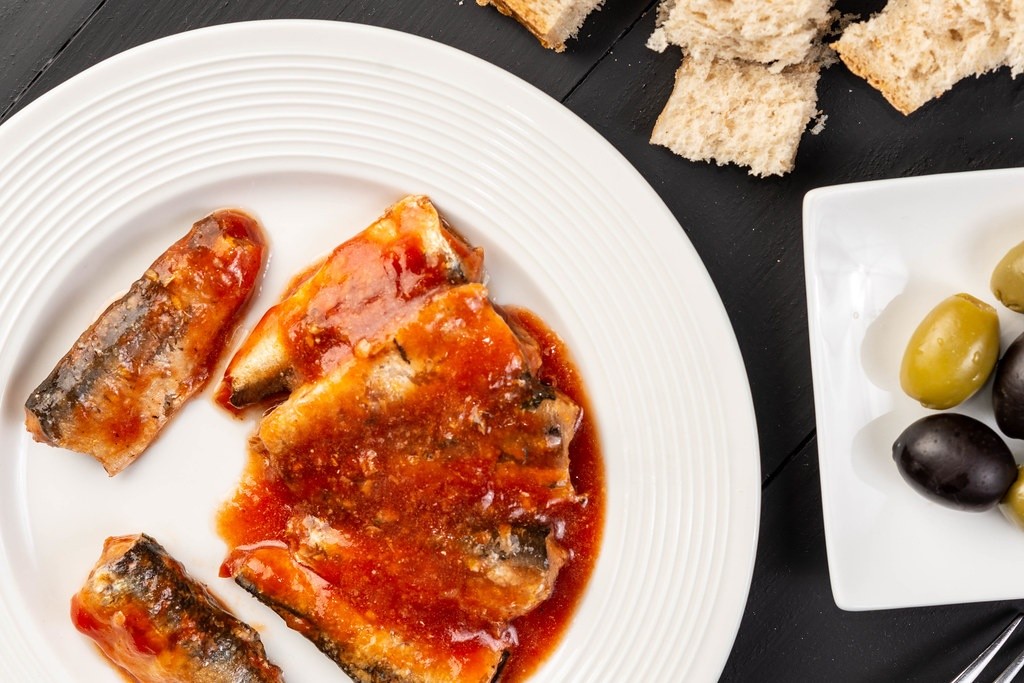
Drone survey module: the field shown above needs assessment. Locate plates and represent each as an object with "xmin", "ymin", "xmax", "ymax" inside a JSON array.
[
  {"xmin": 0, "ymin": 19, "xmax": 763, "ymax": 683},
  {"xmin": 802, "ymin": 167, "xmax": 1024, "ymax": 612}
]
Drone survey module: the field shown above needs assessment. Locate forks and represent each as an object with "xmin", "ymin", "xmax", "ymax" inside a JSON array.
[{"xmin": 949, "ymin": 616, "xmax": 1024, "ymax": 683}]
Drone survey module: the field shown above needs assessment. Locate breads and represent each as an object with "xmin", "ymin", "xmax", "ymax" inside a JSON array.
[{"xmin": 472, "ymin": 0, "xmax": 1024, "ymax": 179}]
